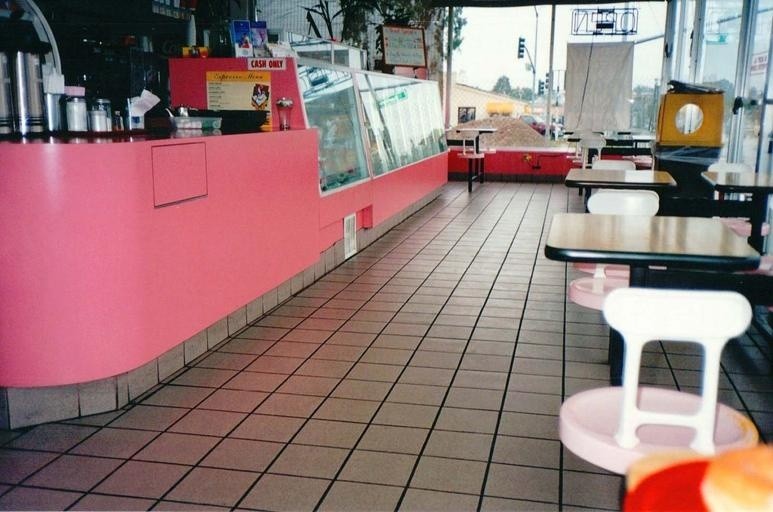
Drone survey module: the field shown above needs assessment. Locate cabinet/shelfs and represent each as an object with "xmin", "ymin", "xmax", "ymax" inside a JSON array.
[{"xmin": 294, "ymin": 57, "xmax": 450, "ymax": 197}]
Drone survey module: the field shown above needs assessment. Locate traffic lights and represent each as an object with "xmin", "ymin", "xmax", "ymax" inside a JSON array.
[
  {"xmin": 545, "ymin": 71, "xmax": 549, "ymax": 88},
  {"xmin": 538, "ymin": 78, "xmax": 544, "ymax": 95},
  {"xmin": 517, "ymin": 36, "xmax": 525, "ymax": 58}
]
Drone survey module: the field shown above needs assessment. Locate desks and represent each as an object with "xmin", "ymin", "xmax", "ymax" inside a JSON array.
[
  {"xmin": 456, "ymin": 129, "xmax": 496, "ymax": 192},
  {"xmin": 545, "ymin": 212, "xmax": 761, "ymax": 386}
]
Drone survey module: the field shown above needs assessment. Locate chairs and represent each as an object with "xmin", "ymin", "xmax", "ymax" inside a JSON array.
[
  {"xmin": 457, "ymin": 127, "xmax": 496, "ymax": 192},
  {"xmin": 560, "ymin": 131, "xmax": 772, "ymax": 215},
  {"xmin": 560, "ymin": 288, "xmax": 759, "ymax": 476}
]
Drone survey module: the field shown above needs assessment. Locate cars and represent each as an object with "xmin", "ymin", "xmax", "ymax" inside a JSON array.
[{"xmin": 518, "ymin": 114, "xmax": 563, "ymax": 135}]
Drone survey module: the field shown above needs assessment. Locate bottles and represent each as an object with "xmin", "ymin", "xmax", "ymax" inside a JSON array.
[
  {"xmin": 66, "ymin": 96, "xmax": 88, "ymax": 132},
  {"xmin": 90, "ymin": 98, "xmax": 113, "ymax": 133},
  {"xmin": 114, "ymin": 111, "xmax": 123, "ymax": 133}
]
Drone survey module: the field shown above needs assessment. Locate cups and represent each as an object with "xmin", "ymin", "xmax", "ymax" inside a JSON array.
[{"xmin": 276, "ymin": 100, "xmax": 294, "ymax": 129}]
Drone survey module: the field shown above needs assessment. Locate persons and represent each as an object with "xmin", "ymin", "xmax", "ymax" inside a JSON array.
[
  {"xmin": 252, "ymin": 29, "xmax": 267, "ymax": 47},
  {"xmin": 238, "ymin": 33, "xmax": 250, "ymax": 48}
]
